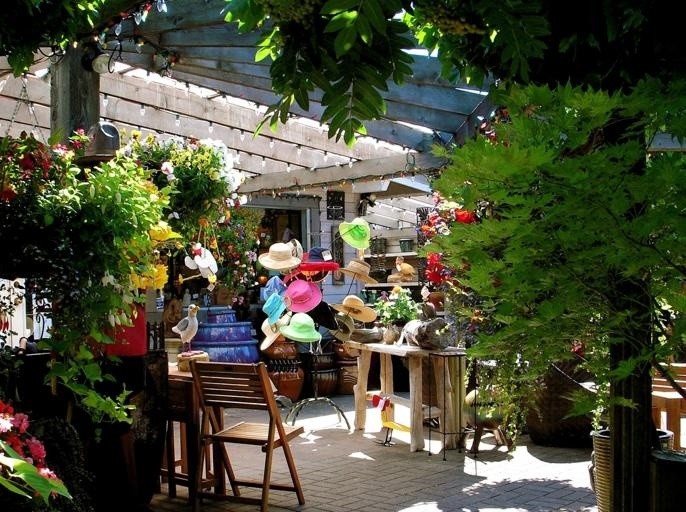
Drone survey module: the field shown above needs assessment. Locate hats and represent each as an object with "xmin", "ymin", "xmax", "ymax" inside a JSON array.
[
  {"xmin": 278, "ymin": 311, "xmax": 323, "ymax": 344},
  {"xmin": 258, "ymin": 311, "xmax": 294, "ymax": 351},
  {"xmin": 258, "ymin": 274, "xmax": 287, "ymax": 302},
  {"xmin": 337, "ymin": 258, "xmax": 378, "ymax": 286},
  {"xmin": 328, "ymin": 311, "xmax": 357, "ymax": 343},
  {"xmin": 261, "ymin": 292, "xmax": 291, "ymax": 326},
  {"xmin": 286, "ymin": 237, "xmax": 304, "ymax": 264},
  {"xmin": 338, "ymin": 217, "xmax": 370, "ymax": 252},
  {"xmin": 332, "ymin": 293, "xmax": 377, "ymax": 323},
  {"xmin": 306, "ymin": 300, "xmax": 340, "ymax": 331},
  {"xmin": 258, "ymin": 242, "xmax": 302, "ymax": 272},
  {"xmin": 297, "ymin": 245, "xmax": 339, "ymax": 274},
  {"xmin": 284, "ymin": 278, "xmax": 324, "ymax": 315}
]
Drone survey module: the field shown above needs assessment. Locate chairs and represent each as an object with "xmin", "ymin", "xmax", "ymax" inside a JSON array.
[{"xmin": 189, "ymin": 359, "xmax": 306, "ymax": 512}]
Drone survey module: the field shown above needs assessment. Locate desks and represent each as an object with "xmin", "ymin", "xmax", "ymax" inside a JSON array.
[{"xmin": 345, "ymin": 339, "xmax": 467, "ymax": 453}]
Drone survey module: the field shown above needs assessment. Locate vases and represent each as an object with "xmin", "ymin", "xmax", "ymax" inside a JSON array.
[
  {"xmin": 210, "ymin": 285, "xmax": 235, "ymax": 305},
  {"xmin": 312, "ymin": 353, "xmax": 335, "ymax": 370},
  {"xmin": 338, "ymin": 360, "xmax": 362, "ymax": 395},
  {"xmin": 333, "ymin": 342, "xmax": 359, "ymax": 361},
  {"xmin": 392, "ymin": 319, "xmax": 409, "ymax": 343},
  {"xmin": 310, "ymin": 370, "xmax": 337, "ymax": 398},
  {"xmin": 266, "ymin": 361, "xmax": 305, "ymax": 403},
  {"xmin": 265, "ymin": 340, "xmax": 298, "ymax": 359}
]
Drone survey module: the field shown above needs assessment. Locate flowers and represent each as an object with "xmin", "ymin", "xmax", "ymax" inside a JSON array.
[
  {"xmin": 205, "ymin": 207, "xmax": 265, "ymax": 304},
  {"xmin": 131, "ymin": 129, "xmax": 230, "ymax": 203},
  {"xmin": 0, "ymin": 131, "xmax": 77, "ymax": 213},
  {"xmin": 421, "ymin": 190, "xmax": 479, "ymax": 286},
  {"xmin": 376, "ymin": 287, "xmax": 419, "ymax": 326},
  {"xmin": 0, "ymin": 401, "xmax": 61, "ymax": 502}
]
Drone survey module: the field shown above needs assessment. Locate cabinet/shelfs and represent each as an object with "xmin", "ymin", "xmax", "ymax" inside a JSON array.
[{"xmin": 363, "ymin": 231, "xmax": 419, "ymax": 286}]
